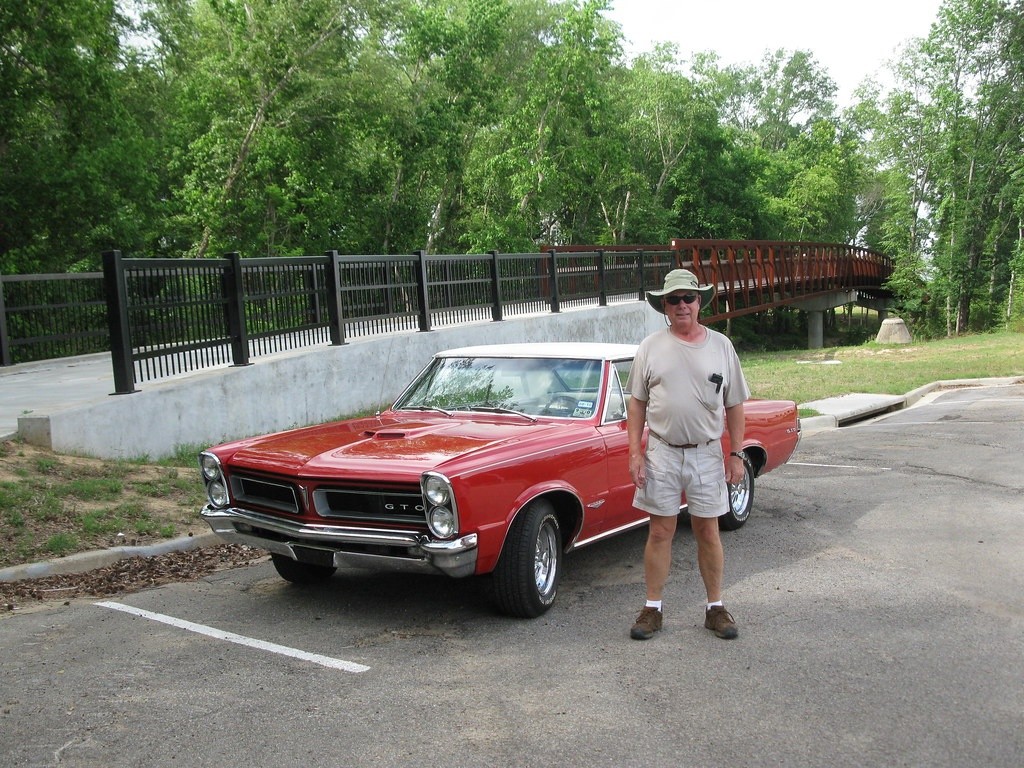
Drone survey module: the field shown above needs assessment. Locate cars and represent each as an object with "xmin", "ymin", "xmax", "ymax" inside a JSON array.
[{"xmin": 199, "ymin": 343, "xmax": 799, "ymax": 622}]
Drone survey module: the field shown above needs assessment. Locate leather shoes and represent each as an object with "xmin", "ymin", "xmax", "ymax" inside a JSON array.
[{"xmin": 649, "ymin": 428, "xmax": 714, "ymax": 448}]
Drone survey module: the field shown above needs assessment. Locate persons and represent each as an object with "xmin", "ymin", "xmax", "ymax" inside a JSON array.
[{"xmin": 625, "ymin": 269, "xmax": 751, "ymax": 640}]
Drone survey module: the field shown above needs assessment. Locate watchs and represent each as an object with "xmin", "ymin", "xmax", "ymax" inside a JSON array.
[{"xmin": 730, "ymin": 450, "xmax": 745, "ymax": 459}]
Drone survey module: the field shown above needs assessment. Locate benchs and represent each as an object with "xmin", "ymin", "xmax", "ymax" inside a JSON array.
[{"xmin": 538, "ymin": 392, "xmax": 630, "ymax": 419}]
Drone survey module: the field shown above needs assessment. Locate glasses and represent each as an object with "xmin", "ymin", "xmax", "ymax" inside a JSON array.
[{"xmin": 663, "ymin": 291, "xmax": 698, "ymax": 305}]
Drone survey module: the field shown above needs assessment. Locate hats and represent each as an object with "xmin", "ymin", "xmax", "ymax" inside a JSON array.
[{"xmin": 646, "ymin": 268, "xmax": 716, "ymax": 315}]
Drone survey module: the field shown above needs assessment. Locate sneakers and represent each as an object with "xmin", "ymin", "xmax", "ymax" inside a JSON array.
[
  {"xmin": 705, "ymin": 605, "xmax": 738, "ymax": 639},
  {"xmin": 631, "ymin": 605, "xmax": 664, "ymax": 639}
]
